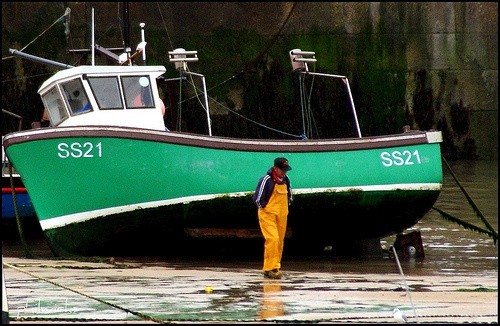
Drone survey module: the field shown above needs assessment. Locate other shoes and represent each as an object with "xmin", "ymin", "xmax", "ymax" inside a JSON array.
[{"xmin": 263, "ymin": 269, "xmax": 282, "ymax": 279}]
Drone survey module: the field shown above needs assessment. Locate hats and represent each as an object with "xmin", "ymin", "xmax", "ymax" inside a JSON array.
[{"xmin": 273, "ymin": 157, "xmax": 292, "ymax": 171}]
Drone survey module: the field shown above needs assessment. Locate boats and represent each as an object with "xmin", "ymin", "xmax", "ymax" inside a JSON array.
[
  {"xmin": 2, "ymin": 162, "xmax": 32, "ymax": 222},
  {"xmin": 2, "ymin": 9, "xmax": 445, "ymax": 257}
]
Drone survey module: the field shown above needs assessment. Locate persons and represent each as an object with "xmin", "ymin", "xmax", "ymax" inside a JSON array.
[{"xmin": 253, "ymin": 157, "xmax": 293, "ymax": 278}]
